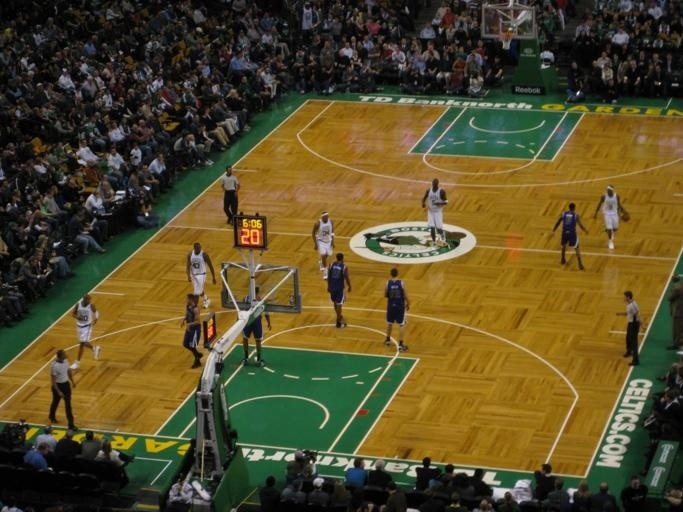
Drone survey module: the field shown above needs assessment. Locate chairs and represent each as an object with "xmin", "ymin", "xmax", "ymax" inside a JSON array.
[
  {"xmin": 284, "ymin": 474, "xmax": 683, "ymax": 512},
  {"xmin": 0, "ymin": 450, "xmax": 129, "ymax": 512}
]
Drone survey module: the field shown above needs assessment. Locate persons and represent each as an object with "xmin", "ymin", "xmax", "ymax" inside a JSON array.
[
  {"xmin": 280, "ymin": 2, "xmax": 683, "ymax": 103},
  {"xmin": 48, "ymin": 348, "xmax": 79, "ymax": 431},
  {"xmin": 312, "ymin": 178, "xmax": 447, "ymax": 351},
  {"xmin": 70, "ymin": 294, "xmax": 100, "ymax": 370},
  {"xmin": 240, "ymin": 288, "xmax": 271, "ymax": 367},
  {"xmin": 552, "ymin": 184, "xmax": 623, "ymax": 270},
  {"xmin": 181, "ymin": 295, "xmax": 204, "ymax": 369},
  {"xmin": 0, "ymin": 1, "xmax": 294, "ymax": 330},
  {"xmin": 614, "ymin": 276, "xmax": 683, "ymax": 446},
  {"xmin": 1, "ymin": 419, "xmax": 683, "ymax": 512}
]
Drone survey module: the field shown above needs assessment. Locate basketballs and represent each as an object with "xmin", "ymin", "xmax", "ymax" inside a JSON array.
[{"xmin": 620, "ymin": 212, "xmax": 630, "ymax": 221}]
